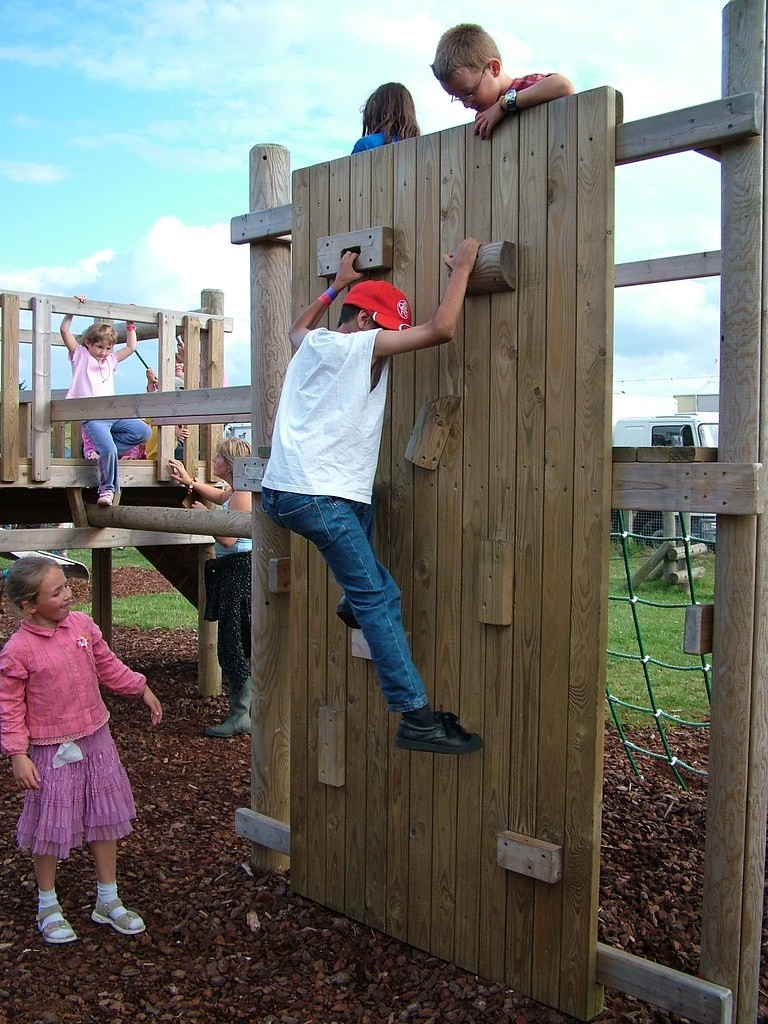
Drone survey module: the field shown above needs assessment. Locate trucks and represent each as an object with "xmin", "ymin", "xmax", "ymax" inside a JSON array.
[{"xmin": 611, "ymin": 394, "xmax": 718, "ymax": 551}]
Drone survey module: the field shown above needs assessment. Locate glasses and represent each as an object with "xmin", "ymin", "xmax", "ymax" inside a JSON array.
[{"xmin": 451, "ymin": 63, "xmax": 489, "ymax": 103}]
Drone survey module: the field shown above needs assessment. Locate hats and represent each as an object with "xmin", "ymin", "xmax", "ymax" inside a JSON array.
[{"xmin": 342, "ymin": 280, "xmax": 412, "ymax": 332}]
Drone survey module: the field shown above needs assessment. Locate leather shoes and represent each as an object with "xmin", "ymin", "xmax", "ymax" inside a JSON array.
[
  {"xmin": 394, "ymin": 705, "xmax": 483, "ymax": 755},
  {"xmin": 336, "ymin": 592, "xmax": 360, "ymax": 629}
]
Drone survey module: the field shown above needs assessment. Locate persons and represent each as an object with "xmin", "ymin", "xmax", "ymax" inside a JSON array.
[
  {"xmin": 169, "ymin": 437, "xmax": 251, "ymax": 737},
  {"xmin": 0, "ymin": 556, "xmax": 163, "ymax": 943},
  {"xmin": 144, "ymin": 342, "xmax": 228, "ymax": 459},
  {"xmin": 261, "ymin": 237, "xmax": 483, "ymax": 755},
  {"xmin": 350, "ymin": 82, "xmax": 420, "ymax": 155},
  {"xmin": 81, "ymin": 427, "xmax": 147, "ymax": 461},
  {"xmin": 60, "ymin": 295, "xmax": 152, "ymax": 506},
  {"xmin": 431, "ymin": 23, "xmax": 574, "ymax": 140}
]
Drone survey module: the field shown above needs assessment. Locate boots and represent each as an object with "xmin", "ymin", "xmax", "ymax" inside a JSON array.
[{"xmin": 206, "ymin": 676, "xmax": 253, "ymax": 737}]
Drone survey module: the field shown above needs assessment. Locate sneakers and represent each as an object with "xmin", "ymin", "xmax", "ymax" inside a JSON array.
[{"xmin": 97, "ymin": 489, "xmax": 115, "ymax": 506}]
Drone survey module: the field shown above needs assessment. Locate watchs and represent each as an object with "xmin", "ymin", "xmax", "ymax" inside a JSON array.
[{"xmin": 505, "ymin": 89, "xmax": 517, "ymax": 111}]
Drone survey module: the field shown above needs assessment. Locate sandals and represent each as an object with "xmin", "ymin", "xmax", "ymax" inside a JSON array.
[
  {"xmin": 35, "ymin": 905, "xmax": 76, "ymax": 943},
  {"xmin": 91, "ymin": 896, "xmax": 145, "ymax": 934}
]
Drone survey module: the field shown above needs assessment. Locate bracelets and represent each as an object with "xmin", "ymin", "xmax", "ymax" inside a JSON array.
[
  {"xmin": 186, "ymin": 476, "xmax": 198, "ymax": 494},
  {"xmin": 318, "ymin": 287, "xmax": 338, "ymax": 308},
  {"xmin": 126, "ymin": 325, "xmax": 135, "ymax": 331},
  {"xmin": 498, "ymin": 96, "xmax": 505, "ymax": 112}
]
